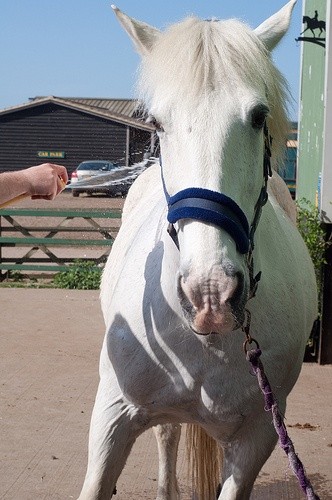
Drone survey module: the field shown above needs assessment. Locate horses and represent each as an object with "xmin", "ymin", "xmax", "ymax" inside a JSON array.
[{"xmin": 76, "ymin": 0, "xmax": 317, "ymax": 500}]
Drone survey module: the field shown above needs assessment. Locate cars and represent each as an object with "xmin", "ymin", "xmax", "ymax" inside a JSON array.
[{"xmin": 70, "ymin": 160, "xmax": 123, "ymax": 198}]
